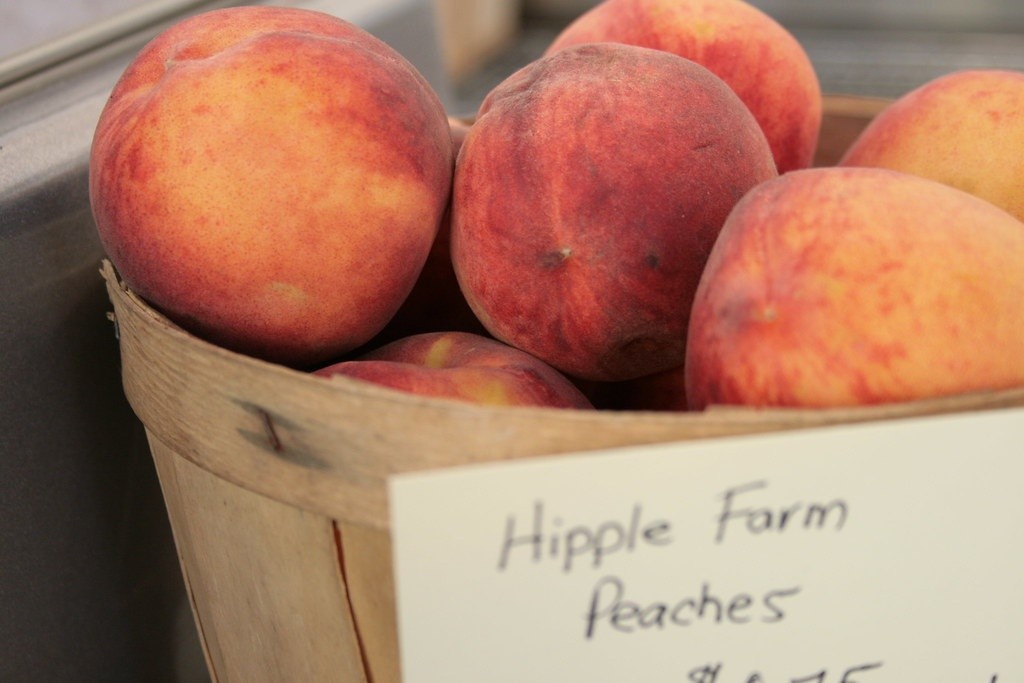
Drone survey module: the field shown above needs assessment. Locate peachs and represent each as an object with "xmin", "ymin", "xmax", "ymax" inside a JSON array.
[{"xmin": 88, "ymin": 0, "xmax": 1024, "ymax": 410}]
